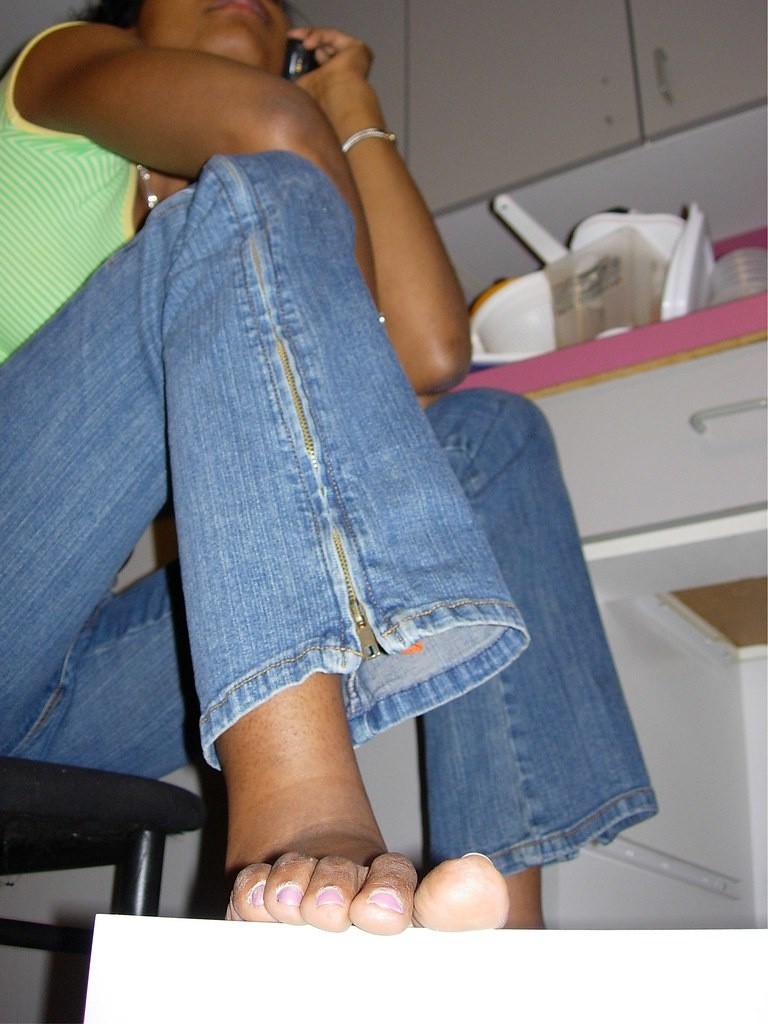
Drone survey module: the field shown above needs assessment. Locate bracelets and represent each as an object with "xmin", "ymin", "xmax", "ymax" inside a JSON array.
[{"xmin": 341, "ymin": 128, "xmax": 396, "ymax": 153}]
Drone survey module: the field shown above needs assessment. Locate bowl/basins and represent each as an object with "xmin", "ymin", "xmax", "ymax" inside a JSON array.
[
  {"xmin": 465, "ymin": 271, "xmax": 551, "ymax": 363},
  {"xmin": 568, "ymin": 211, "xmax": 687, "ymax": 264}
]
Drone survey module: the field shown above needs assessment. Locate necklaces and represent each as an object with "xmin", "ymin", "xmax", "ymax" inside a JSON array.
[{"xmin": 136, "ymin": 165, "xmax": 159, "ymax": 210}]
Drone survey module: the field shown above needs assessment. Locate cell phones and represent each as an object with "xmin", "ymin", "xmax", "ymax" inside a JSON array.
[{"xmin": 283, "ymin": 39, "xmax": 313, "ymax": 82}]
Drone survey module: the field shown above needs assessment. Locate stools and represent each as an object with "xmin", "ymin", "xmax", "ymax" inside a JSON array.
[{"xmin": 0, "ymin": 746, "xmax": 231, "ymax": 1024}]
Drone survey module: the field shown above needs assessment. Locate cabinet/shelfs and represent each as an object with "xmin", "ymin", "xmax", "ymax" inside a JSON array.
[
  {"xmin": 278, "ymin": 0, "xmax": 768, "ymax": 309},
  {"xmin": 332, "ymin": 338, "xmax": 768, "ymax": 929}
]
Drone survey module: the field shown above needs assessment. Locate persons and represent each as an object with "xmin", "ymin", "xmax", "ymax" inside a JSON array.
[{"xmin": 1, "ymin": 0, "xmax": 656, "ymax": 937}]
